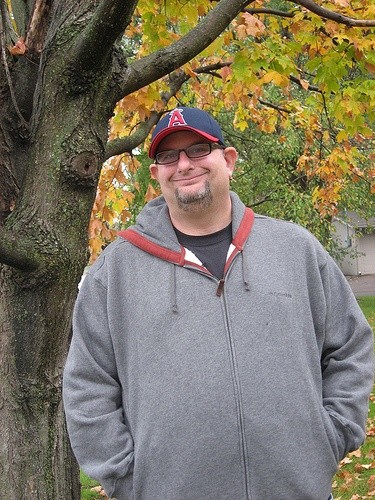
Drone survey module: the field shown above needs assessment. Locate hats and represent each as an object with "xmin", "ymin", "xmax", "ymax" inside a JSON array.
[{"xmin": 149, "ymin": 105, "xmax": 224, "ymax": 160}]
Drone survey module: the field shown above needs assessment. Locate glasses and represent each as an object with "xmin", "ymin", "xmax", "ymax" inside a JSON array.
[{"xmin": 154, "ymin": 141, "xmax": 225, "ymax": 165}]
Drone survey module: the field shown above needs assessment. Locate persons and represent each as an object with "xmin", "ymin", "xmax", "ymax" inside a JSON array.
[{"xmin": 60, "ymin": 105, "xmax": 374, "ymax": 500}]
